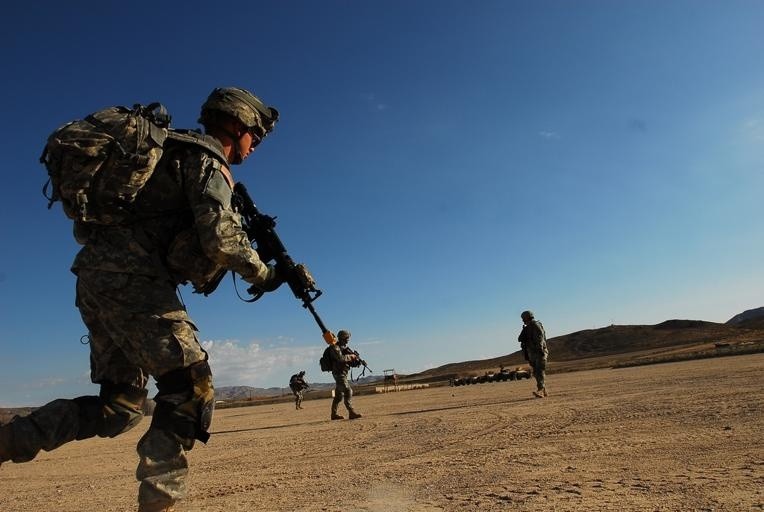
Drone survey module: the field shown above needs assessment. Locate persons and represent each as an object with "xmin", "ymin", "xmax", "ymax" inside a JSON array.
[
  {"xmin": 518, "ymin": 311, "xmax": 549, "ymax": 398},
  {"xmin": 1, "ymin": 88, "xmax": 290, "ymax": 511},
  {"xmin": 320, "ymin": 330, "xmax": 362, "ymax": 420},
  {"xmin": 289, "ymin": 371, "xmax": 310, "ymax": 410}
]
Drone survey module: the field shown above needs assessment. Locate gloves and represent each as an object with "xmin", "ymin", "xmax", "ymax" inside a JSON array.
[{"xmin": 255, "ymin": 264, "xmax": 284, "ymax": 292}]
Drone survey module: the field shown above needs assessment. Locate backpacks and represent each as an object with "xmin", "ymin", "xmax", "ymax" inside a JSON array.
[
  {"xmin": 289, "ymin": 374, "xmax": 299, "ymax": 392},
  {"xmin": 39, "ymin": 102, "xmax": 231, "ymax": 227},
  {"xmin": 318, "ymin": 347, "xmax": 332, "ymax": 373}
]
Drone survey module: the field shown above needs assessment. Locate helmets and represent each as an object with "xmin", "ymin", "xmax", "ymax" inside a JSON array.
[
  {"xmin": 337, "ymin": 330, "xmax": 351, "ymax": 339},
  {"xmin": 197, "ymin": 86, "xmax": 280, "ymax": 147},
  {"xmin": 521, "ymin": 310, "xmax": 534, "ymax": 322}
]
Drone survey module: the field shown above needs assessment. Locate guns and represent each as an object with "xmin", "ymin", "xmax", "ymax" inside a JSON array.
[
  {"xmin": 298, "ymin": 378, "xmax": 310, "ymax": 388},
  {"xmin": 233, "ymin": 181, "xmax": 335, "ymax": 347},
  {"xmin": 343, "ymin": 347, "xmax": 372, "ymax": 373}
]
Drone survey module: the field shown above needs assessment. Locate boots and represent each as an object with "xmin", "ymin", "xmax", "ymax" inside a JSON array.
[
  {"xmin": 533, "ymin": 386, "xmax": 548, "ymax": 398},
  {"xmin": 331, "ymin": 414, "xmax": 344, "ymax": 420},
  {"xmin": 349, "ymin": 408, "xmax": 361, "ymax": 419}
]
